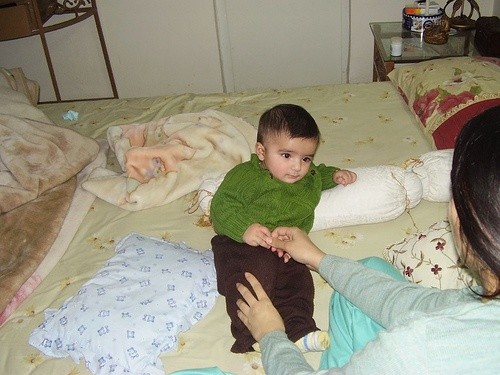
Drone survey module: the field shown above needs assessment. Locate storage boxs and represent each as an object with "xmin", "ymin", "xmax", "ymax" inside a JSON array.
[{"xmin": 402, "ymin": 7, "xmax": 446, "ymax": 32}]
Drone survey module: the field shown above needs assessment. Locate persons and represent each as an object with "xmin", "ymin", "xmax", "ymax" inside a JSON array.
[
  {"xmin": 236, "ymin": 106, "xmax": 500, "ymax": 375},
  {"xmin": 209, "ymin": 103, "xmax": 357, "ymax": 351}
]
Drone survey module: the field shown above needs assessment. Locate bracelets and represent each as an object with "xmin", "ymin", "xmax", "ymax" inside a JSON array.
[{"xmin": 334, "ymin": 169, "xmax": 340, "ymax": 175}]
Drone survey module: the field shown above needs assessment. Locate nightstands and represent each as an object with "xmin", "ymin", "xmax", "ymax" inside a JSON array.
[{"xmin": 370, "ymin": 23, "xmax": 484, "ymax": 82}]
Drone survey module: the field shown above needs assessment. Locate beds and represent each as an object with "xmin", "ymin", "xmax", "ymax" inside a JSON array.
[{"xmin": 0, "ymin": 82, "xmax": 499, "ymax": 375}]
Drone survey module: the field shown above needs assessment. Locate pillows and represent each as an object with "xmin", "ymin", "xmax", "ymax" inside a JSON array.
[
  {"xmin": 385, "ymin": 219, "xmax": 479, "ymax": 289},
  {"xmin": 29, "ymin": 233, "xmax": 219, "ymax": 375},
  {"xmin": 388, "ymin": 56, "xmax": 500, "ymax": 150}
]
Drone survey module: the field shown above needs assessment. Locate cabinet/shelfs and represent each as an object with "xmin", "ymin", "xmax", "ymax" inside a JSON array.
[{"xmin": 0, "ymin": 0, "xmax": 118, "ymax": 103}]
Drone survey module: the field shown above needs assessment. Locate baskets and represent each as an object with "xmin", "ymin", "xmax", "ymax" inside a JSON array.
[
  {"xmin": 444, "ymin": 0, "xmax": 480, "ymax": 31},
  {"xmin": 423, "ymin": 19, "xmax": 449, "ymax": 45}
]
